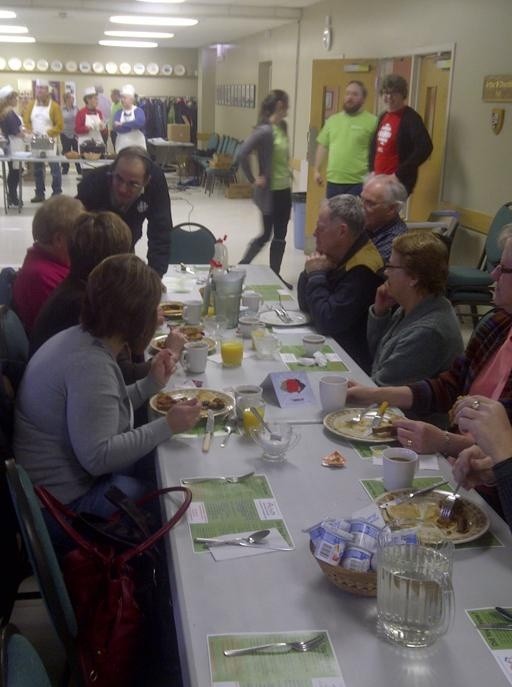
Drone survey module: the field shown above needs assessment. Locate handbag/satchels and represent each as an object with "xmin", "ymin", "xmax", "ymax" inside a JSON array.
[{"xmin": 32, "ymin": 484, "xmax": 193, "ymax": 685}]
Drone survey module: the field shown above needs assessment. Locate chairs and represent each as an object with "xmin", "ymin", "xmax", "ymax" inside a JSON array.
[
  {"xmin": 191, "ymin": 133, "xmax": 244, "ymax": 197},
  {"xmin": 0, "ymin": 199, "xmax": 511, "ymax": 686}
]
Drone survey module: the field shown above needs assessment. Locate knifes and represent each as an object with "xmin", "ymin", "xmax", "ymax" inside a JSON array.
[
  {"xmin": 193, "ymin": 536, "xmax": 296, "ymax": 551},
  {"xmin": 364, "ymin": 400, "xmax": 389, "ymax": 437},
  {"xmin": 274, "ymin": 306, "xmax": 291, "ymax": 323},
  {"xmin": 380, "ymin": 477, "xmax": 448, "ymax": 509},
  {"xmin": 477, "ymin": 621, "xmax": 511, "ymax": 630},
  {"xmin": 203, "ymin": 409, "xmax": 214, "ymax": 452}
]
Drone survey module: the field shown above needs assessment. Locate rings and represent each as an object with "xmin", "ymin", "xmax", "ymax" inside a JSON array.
[
  {"xmin": 407, "ymin": 439, "xmax": 413, "ymax": 447},
  {"xmin": 471, "ymin": 399, "xmax": 480, "ymax": 410}
]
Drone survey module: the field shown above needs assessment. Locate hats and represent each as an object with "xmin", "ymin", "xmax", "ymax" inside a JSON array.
[
  {"xmin": 0, "ymin": 86, "xmax": 13, "ymax": 98},
  {"xmin": 35, "ymin": 79, "xmax": 49, "ymax": 87},
  {"xmin": 83, "ymin": 87, "xmax": 96, "ymax": 97},
  {"xmin": 120, "ymin": 85, "xmax": 135, "ymax": 96}
]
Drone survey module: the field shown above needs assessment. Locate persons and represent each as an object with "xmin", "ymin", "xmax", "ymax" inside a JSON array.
[
  {"xmin": 73, "ymin": 145, "xmax": 174, "ymax": 292},
  {"xmin": 446, "ymin": 389, "xmax": 512, "ymax": 533},
  {"xmin": 6, "ymin": 254, "xmax": 204, "ymax": 577},
  {"xmin": 311, "ymin": 78, "xmax": 380, "ymax": 202},
  {"xmin": 0, "ymin": 78, "xmax": 149, "ymax": 204},
  {"xmin": 26, "ymin": 211, "xmax": 139, "ymax": 365},
  {"xmin": 338, "ymin": 221, "xmax": 512, "ymax": 471},
  {"xmin": 233, "ymin": 85, "xmax": 296, "ymax": 293},
  {"xmin": 10, "ymin": 193, "xmax": 88, "ymax": 334},
  {"xmin": 297, "ymin": 193, "xmax": 384, "ymax": 374},
  {"xmin": 355, "ymin": 169, "xmax": 407, "ymax": 268},
  {"xmin": 372, "ymin": 73, "xmax": 431, "ymax": 212},
  {"xmin": 363, "ymin": 224, "xmax": 467, "ymax": 421}
]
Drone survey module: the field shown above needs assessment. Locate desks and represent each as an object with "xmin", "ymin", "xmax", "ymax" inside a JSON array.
[
  {"xmin": 147, "ymin": 137, "xmax": 195, "ymax": 174},
  {"xmin": 0, "ymin": 151, "xmax": 115, "ymax": 214}
]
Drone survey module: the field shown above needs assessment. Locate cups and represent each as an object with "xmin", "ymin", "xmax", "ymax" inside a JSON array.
[
  {"xmin": 382, "ymin": 447, "xmax": 418, "ymax": 489},
  {"xmin": 240, "ymin": 397, "xmax": 265, "ymax": 436},
  {"xmin": 204, "ymin": 290, "xmax": 280, "ymax": 367},
  {"xmin": 211, "ymin": 271, "xmax": 246, "ymax": 328},
  {"xmin": 375, "ymin": 518, "xmax": 456, "ymax": 649},
  {"xmin": 253, "ymin": 422, "xmax": 301, "ymax": 460},
  {"xmin": 302, "ymin": 334, "xmax": 325, "ymax": 356},
  {"xmin": 234, "ymin": 383, "xmax": 263, "ymax": 415},
  {"xmin": 320, "ymin": 375, "xmax": 352, "ymax": 413},
  {"xmin": 182, "ymin": 341, "xmax": 209, "ymax": 373},
  {"xmin": 182, "ymin": 300, "xmax": 202, "ymax": 324},
  {"xmin": 306, "ymin": 516, "xmax": 422, "ymax": 573}
]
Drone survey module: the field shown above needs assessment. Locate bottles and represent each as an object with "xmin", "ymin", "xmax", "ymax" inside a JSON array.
[{"xmin": 212, "ymin": 234, "xmax": 229, "ymax": 270}]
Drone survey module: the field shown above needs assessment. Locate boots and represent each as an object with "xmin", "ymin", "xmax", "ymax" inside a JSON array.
[
  {"xmin": 239, "ymin": 240, "xmax": 265, "ymax": 265},
  {"xmin": 269, "ymin": 240, "xmax": 293, "ymax": 289}
]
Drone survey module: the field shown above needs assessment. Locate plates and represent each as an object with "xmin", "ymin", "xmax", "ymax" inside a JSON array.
[
  {"xmin": 150, "ymin": 388, "xmax": 235, "ymax": 418},
  {"xmin": 158, "ymin": 300, "xmax": 186, "ymax": 316},
  {"xmin": 323, "ymin": 408, "xmax": 407, "ymax": 444},
  {"xmin": 151, "ymin": 334, "xmax": 218, "ymax": 353},
  {"xmin": 375, "ymin": 487, "xmax": 490, "ymax": 544},
  {"xmin": 0, "ymin": 58, "xmax": 186, "ymax": 76},
  {"xmin": 256, "ymin": 310, "xmax": 312, "ymax": 328}
]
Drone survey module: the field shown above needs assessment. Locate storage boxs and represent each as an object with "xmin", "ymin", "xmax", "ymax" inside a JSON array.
[
  {"xmin": 230, "ymin": 184, "xmax": 253, "ymax": 198},
  {"xmin": 167, "ymin": 123, "xmax": 190, "ymax": 142}
]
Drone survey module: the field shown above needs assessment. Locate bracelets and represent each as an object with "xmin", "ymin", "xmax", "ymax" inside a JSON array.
[{"xmin": 439, "ymin": 430, "xmax": 450, "ymax": 453}]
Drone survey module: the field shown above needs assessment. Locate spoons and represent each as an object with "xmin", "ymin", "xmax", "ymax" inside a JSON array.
[
  {"xmin": 221, "ymin": 411, "xmax": 238, "ymax": 449},
  {"xmin": 250, "ymin": 407, "xmax": 283, "ymax": 441},
  {"xmin": 206, "ymin": 529, "xmax": 270, "ymax": 547}
]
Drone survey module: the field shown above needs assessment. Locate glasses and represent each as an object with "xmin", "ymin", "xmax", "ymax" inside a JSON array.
[
  {"xmin": 383, "ymin": 89, "xmax": 400, "ymax": 96},
  {"xmin": 114, "ymin": 175, "xmax": 151, "ymax": 193},
  {"xmin": 496, "ymin": 265, "xmax": 511, "ymax": 273},
  {"xmin": 383, "ymin": 262, "xmax": 403, "ymax": 272}
]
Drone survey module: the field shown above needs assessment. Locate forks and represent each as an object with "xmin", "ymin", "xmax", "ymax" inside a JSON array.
[
  {"xmin": 346, "ymin": 401, "xmax": 378, "ymax": 423},
  {"xmin": 440, "ymin": 468, "xmax": 472, "ymax": 516},
  {"xmin": 183, "ymin": 470, "xmax": 255, "ymax": 485},
  {"xmin": 222, "ymin": 631, "xmax": 326, "ymax": 657}
]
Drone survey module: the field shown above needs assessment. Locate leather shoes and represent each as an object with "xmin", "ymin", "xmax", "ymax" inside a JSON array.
[{"xmin": 31, "ymin": 195, "xmax": 45, "ymax": 203}]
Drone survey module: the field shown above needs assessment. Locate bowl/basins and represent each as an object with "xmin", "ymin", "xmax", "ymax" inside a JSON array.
[
  {"xmin": 65, "ymin": 152, "xmax": 81, "ymax": 159},
  {"xmin": 84, "ymin": 151, "xmax": 102, "ymax": 160}
]
